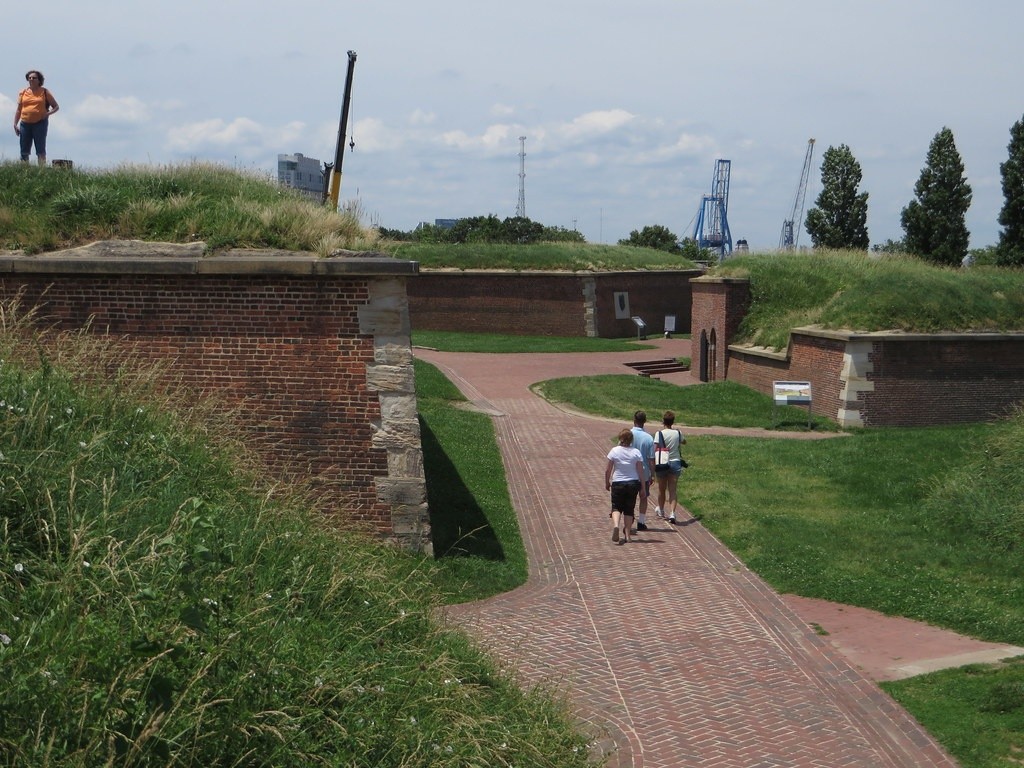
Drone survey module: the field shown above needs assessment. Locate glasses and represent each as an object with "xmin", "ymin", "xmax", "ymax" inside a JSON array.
[{"xmin": 27, "ymin": 77, "xmax": 39, "ymax": 80}]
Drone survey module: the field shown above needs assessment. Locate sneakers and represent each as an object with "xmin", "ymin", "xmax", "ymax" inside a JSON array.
[
  {"xmin": 655, "ymin": 505, "xmax": 664, "ymax": 517},
  {"xmin": 667, "ymin": 512, "xmax": 676, "ymax": 525}
]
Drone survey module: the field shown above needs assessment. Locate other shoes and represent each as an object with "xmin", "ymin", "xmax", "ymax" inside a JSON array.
[
  {"xmin": 637, "ymin": 522, "xmax": 648, "ymax": 530},
  {"xmin": 624, "ymin": 537, "xmax": 633, "ymax": 544},
  {"xmin": 624, "ymin": 525, "xmax": 639, "ymax": 535},
  {"xmin": 610, "ymin": 526, "xmax": 620, "ymax": 544}
]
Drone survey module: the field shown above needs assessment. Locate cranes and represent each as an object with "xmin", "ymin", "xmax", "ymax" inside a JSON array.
[
  {"xmin": 324, "ymin": 49, "xmax": 359, "ymax": 214},
  {"xmin": 668, "ymin": 157, "xmax": 735, "ymax": 254},
  {"xmin": 777, "ymin": 138, "xmax": 816, "ymax": 257}
]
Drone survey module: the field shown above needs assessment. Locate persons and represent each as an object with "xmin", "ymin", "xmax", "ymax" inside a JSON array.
[
  {"xmin": 13, "ymin": 71, "xmax": 60, "ymax": 166},
  {"xmin": 605, "ymin": 429, "xmax": 645, "ymax": 542},
  {"xmin": 618, "ymin": 410, "xmax": 655, "ymax": 531},
  {"xmin": 653, "ymin": 410, "xmax": 690, "ymax": 524}
]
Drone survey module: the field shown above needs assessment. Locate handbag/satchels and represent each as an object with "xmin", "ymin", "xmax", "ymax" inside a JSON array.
[{"xmin": 651, "ymin": 432, "xmax": 671, "ymax": 470}]
